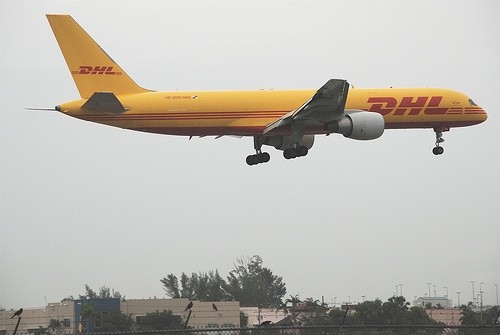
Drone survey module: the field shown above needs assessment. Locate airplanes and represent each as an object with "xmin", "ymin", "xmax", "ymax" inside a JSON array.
[{"xmin": 24, "ymin": 14, "xmax": 488, "ymax": 165}]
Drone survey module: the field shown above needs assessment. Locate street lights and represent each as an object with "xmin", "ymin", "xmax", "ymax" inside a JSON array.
[{"xmin": 393, "ymin": 280, "xmax": 499, "ymax": 326}]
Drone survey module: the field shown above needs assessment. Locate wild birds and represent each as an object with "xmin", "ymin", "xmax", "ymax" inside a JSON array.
[
  {"xmin": 11, "ymin": 308, "xmax": 23, "ymax": 319},
  {"xmin": 184, "ymin": 302, "xmax": 193, "ymax": 311},
  {"xmin": 212, "ymin": 304, "xmax": 217, "ymax": 311}
]
